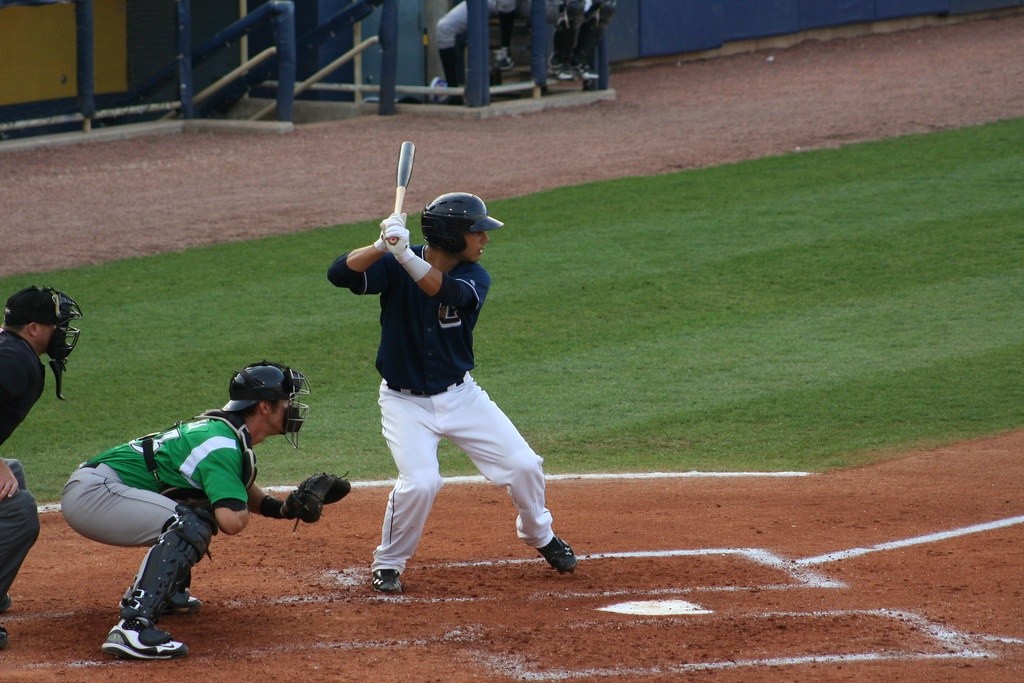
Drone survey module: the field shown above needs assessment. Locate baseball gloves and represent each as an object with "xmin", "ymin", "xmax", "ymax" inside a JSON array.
[{"xmin": 282, "ymin": 472, "xmax": 351, "ymax": 523}]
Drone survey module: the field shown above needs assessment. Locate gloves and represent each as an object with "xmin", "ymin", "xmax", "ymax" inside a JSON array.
[
  {"xmin": 383, "ymin": 226, "xmax": 415, "ymax": 264},
  {"xmin": 373, "ymin": 212, "xmax": 407, "ymax": 253}
]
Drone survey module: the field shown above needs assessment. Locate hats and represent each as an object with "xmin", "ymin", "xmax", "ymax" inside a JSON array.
[{"xmin": 5, "ymin": 287, "xmax": 68, "ymax": 326}]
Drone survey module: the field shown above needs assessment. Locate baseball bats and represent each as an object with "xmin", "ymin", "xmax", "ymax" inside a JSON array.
[{"xmin": 387, "ymin": 141, "xmax": 415, "ymax": 246}]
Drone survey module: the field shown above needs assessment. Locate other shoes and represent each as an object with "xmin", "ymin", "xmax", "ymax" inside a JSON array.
[
  {"xmin": 0, "ymin": 593, "xmax": 12, "ymax": 612},
  {"xmin": 0, "ymin": 626, "xmax": 11, "ymax": 648}
]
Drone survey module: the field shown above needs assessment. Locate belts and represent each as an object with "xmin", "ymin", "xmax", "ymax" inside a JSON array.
[
  {"xmin": 387, "ymin": 378, "xmax": 464, "ymax": 397},
  {"xmin": 81, "ymin": 462, "xmax": 98, "ymax": 468}
]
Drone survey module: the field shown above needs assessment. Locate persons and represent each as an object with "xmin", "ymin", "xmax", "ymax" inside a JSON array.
[
  {"xmin": 0, "ymin": 284, "xmax": 83, "ymax": 650},
  {"xmin": 61, "ymin": 360, "xmax": 352, "ymax": 661},
  {"xmin": 437, "ymin": 0, "xmax": 617, "ymax": 106},
  {"xmin": 326, "ymin": 192, "xmax": 578, "ymax": 595}
]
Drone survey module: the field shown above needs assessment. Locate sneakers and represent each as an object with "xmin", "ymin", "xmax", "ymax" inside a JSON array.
[
  {"xmin": 538, "ymin": 534, "xmax": 577, "ymax": 571},
  {"xmin": 102, "ymin": 617, "xmax": 188, "ymax": 659},
  {"xmin": 372, "ymin": 569, "xmax": 402, "ymax": 593},
  {"xmin": 493, "ymin": 47, "xmax": 515, "ymax": 70},
  {"xmin": 547, "ymin": 52, "xmax": 573, "ymax": 80},
  {"xmin": 573, "ymin": 63, "xmax": 599, "ymax": 80},
  {"xmin": 160, "ymin": 596, "xmax": 202, "ymax": 614}
]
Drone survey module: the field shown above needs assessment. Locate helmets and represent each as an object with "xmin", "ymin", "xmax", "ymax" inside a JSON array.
[
  {"xmin": 222, "ymin": 365, "xmax": 295, "ymax": 412},
  {"xmin": 421, "ymin": 192, "xmax": 504, "ymax": 253}
]
